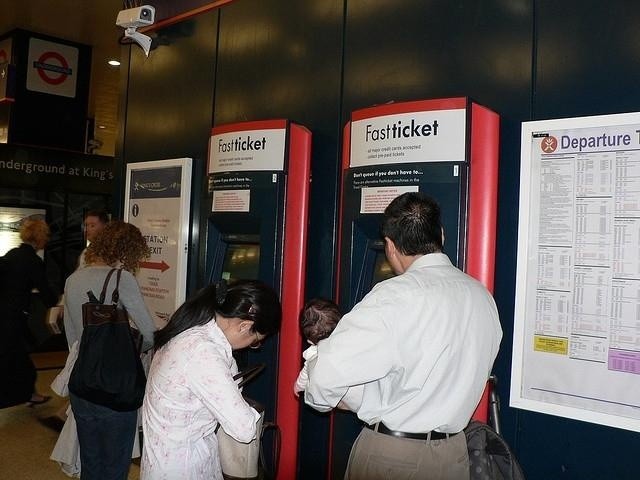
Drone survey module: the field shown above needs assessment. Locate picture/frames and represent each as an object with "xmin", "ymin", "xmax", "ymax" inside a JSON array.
[{"xmin": 507, "ymin": 111, "xmax": 640, "ymax": 436}]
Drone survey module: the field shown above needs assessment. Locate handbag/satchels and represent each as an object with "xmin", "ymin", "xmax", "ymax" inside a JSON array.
[
  {"xmin": 67, "ymin": 268, "xmax": 146, "ymax": 413},
  {"xmin": 213, "ymin": 363, "xmax": 269, "ymax": 479}
]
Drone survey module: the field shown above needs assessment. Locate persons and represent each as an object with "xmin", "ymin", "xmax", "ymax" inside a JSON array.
[
  {"xmin": 293, "ymin": 300, "xmax": 350, "ymax": 410},
  {"xmin": 48, "ymin": 220, "xmax": 155, "ymax": 479},
  {"xmin": 140, "ymin": 273, "xmax": 286, "ymax": 479},
  {"xmin": 0, "ymin": 213, "xmax": 62, "ymax": 402},
  {"xmin": 84, "ymin": 208, "xmax": 111, "ymax": 242},
  {"xmin": 304, "ymin": 192, "xmax": 504, "ymax": 479},
  {"xmin": 48, "ymin": 248, "xmax": 141, "ymax": 477}
]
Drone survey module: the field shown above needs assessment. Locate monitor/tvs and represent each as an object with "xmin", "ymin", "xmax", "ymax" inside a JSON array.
[
  {"xmin": 220, "ymin": 243, "xmax": 261, "ymax": 285},
  {"xmin": 370, "ymin": 252, "xmax": 398, "ymax": 291}
]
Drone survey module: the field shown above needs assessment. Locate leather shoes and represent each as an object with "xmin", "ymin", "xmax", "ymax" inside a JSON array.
[{"xmin": 28, "ymin": 393, "xmax": 51, "ymax": 405}]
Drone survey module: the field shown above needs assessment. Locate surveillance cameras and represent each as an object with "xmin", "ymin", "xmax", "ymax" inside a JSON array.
[{"xmin": 116, "ymin": 4, "xmax": 156, "ymax": 29}]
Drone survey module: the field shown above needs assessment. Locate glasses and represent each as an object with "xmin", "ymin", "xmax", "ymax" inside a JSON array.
[{"xmin": 248, "ymin": 329, "xmax": 264, "ymax": 350}]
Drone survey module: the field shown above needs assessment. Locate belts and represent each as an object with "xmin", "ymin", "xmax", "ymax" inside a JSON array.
[{"xmin": 363, "ymin": 420, "xmax": 460, "ymax": 441}]
